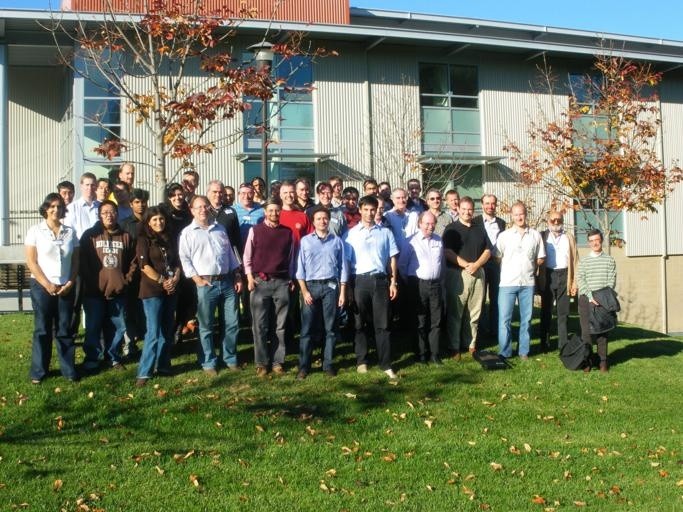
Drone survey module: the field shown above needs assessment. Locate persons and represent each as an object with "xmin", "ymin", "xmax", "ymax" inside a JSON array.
[
  {"xmin": 539, "ymin": 213, "xmax": 580, "ymax": 353},
  {"xmin": 576, "ymin": 229, "xmax": 616, "ymax": 374},
  {"xmin": 23, "ymin": 164, "xmax": 546, "ymax": 386}
]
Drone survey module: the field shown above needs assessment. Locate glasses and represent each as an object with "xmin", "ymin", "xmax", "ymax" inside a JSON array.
[{"xmin": 551, "ymin": 217, "xmax": 562, "ymax": 224}]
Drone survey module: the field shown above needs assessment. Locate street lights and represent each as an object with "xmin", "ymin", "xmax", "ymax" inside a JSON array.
[{"xmin": 250, "ymin": 37, "xmax": 281, "ymax": 193}]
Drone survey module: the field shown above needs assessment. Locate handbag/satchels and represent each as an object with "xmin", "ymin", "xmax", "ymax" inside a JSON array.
[{"xmin": 559, "ymin": 334, "xmax": 591, "ymax": 371}]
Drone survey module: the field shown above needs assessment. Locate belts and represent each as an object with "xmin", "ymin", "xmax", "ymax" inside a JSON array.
[
  {"xmin": 200, "ymin": 275, "xmax": 226, "ymax": 280},
  {"xmin": 306, "ymin": 279, "xmax": 338, "ymax": 284}
]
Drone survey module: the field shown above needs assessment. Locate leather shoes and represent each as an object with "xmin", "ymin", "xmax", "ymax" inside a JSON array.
[
  {"xmin": 600, "ymin": 366, "xmax": 608, "ymax": 374},
  {"xmin": 256, "ymin": 367, "xmax": 267, "ymax": 377},
  {"xmin": 415, "ymin": 354, "xmax": 427, "ymax": 364},
  {"xmin": 385, "ymin": 369, "xmax": 397, "ymax": 379},
  {"xmin": 297, "ymin": 371, "xmax": 309, "ymax": 380},
  {"xmin": 431, "ymin": 355, "xmax": 442, "ymax": 363},
  {"xmin": 584, "ymin": 366, "xmax": 590, "ymax": 372},
  {"xmin": 327, "ymin": 370, "xmax": 338, "ymax": 376},
  {"xmin": 136, "ymin": 379, "xmax": 145, "ymax": 387},
  {"xmin": 357, "ymin": 364, "xmax": 367, "ymax": 373},
  {"xmin": 207, "ymin": 369, "xmax": 217, "ymax": 376},
  {"xmin": 230, "ymin": 366, "xmax": 241, "ymax": 372},
  {"xmin": 272, "ymin": 367, "xmax": 285, "ymax": 374}
]
